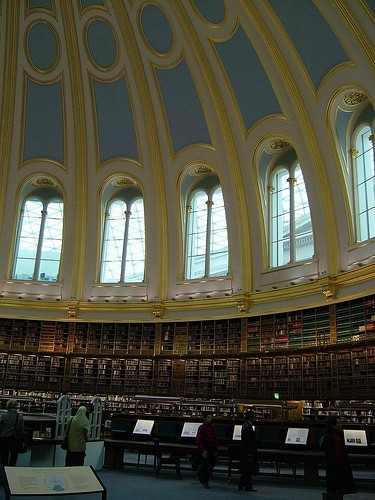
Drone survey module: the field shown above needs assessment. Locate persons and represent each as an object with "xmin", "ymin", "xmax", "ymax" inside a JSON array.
[
  {"xmin": 0, "ymin": 399, "xmax": 24, "ymax": 486},
  {"xmin": 324, "ymin": 415, "xmax": 357, "ymax": 500},
  {"xmin": 195, "ymin": 413, "xmax": 219, "ymax": 489},
  {"xmin": 65, "ymin": 406, "xmax": 90, "ymax": 467},
  {"xmin": 238, "ymin": 411, "xmax": 257, "ymax": 492}
]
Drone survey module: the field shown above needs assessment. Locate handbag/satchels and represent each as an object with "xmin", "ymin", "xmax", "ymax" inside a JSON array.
[
  {"xmin": 9, "ymin": 413, "xmax": 28, "ymax": 453},
  {"xmin": 61, "ymin": 416, "xmax": 74, "ymax": 450}
]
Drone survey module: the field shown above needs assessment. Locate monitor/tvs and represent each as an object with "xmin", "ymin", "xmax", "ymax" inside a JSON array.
[
  {"xmin": 132, "ymin": 418, "xmax": 155, "ymax": 437},
  {"xmin": 180, "ymin": 421, "xmax": 203, "ymax": 438},
  {"xmin": 232, "ymin": 425, "xmax": 256, "ymax": 442},
  {"xmin": 284, "ymin": 427, "xmax": 310, "ymax": 445},
  {"xmin": 343, "ymin": 429, "xmax": 368, "ymax": 447}
]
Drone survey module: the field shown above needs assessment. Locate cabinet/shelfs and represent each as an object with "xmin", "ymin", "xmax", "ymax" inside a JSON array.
[{"xmin": 0, "ymin": 293, "xmax": 375, "ymax": 423}]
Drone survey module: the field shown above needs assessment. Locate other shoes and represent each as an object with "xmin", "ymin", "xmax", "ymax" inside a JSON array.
[
  {"xmin": 203, "ymin": 482, "xmax": 210, "ymax": 489},
  {"xmin": 238, "ymin": 481, "xmax": 257, "ymax": 492}
]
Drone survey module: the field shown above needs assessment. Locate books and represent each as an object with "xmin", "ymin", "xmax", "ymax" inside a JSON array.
[
  {"xmin": 0, "ymin": 318, "xmax": 68, "ymax": 353},
  {"xmin": 247, "ymin": 306, "xmax": 330, "ymax": 354},
  {"xmin": 338, "ymin": 299, "xmax": 375, "ymax": 342},
  {"xmin": 333, "ymin": 349, "xmax": 375, "ymax": 425},
  {"xmin": 240, "ymin": 351, "xmax": 331, "ymax": 421},
  {"xmin": 154, "ymin": 359, "xmax": 240, "ymax": 417},
  {"xmin": 0, "ymin": 353, "xmax": 65, "ymax": 413},
  {"xmin": 65, "ymin": 357, "xmax": 154, "ymax": 414},
  {"xmin": 70, "ymin": 322, "xmax": 156, "ymax": 350},
  {"xmin": 160, "ymin": 318, "xmax": 241, "ymax": 350}
]
